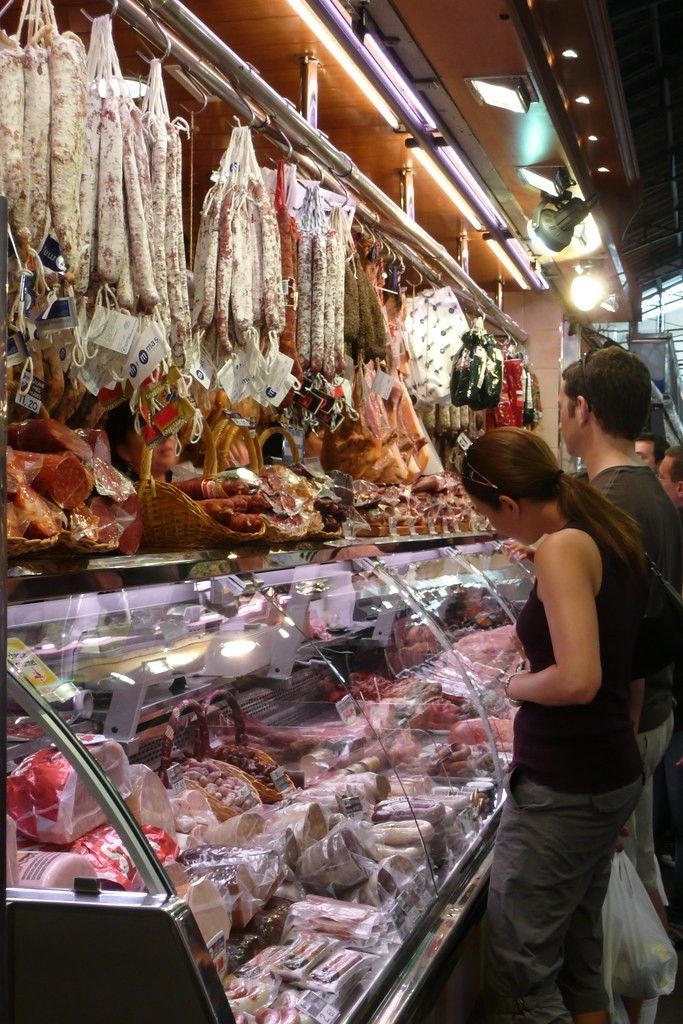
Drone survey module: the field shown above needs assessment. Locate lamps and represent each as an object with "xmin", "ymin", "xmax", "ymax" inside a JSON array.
[
  {"xmin": 527, "ymin": 190, "xmax": 600, "ymax": 257},
  {"xmin": 471, "ymin": 77, "xmax": 531, "ymax": 114},
  {"xmin": 518, "ymin": 167, "xmax": 569, "ymax": 197}
]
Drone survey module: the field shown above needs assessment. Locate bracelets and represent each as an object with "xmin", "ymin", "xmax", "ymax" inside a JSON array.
[{"xmin": 504, "ymin": 672, "xmax": 522, "ymax": 703}]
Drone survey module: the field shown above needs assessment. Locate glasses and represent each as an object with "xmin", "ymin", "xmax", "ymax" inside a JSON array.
[
  {"xmin": 461, "ymin": 449, "xmax": 498, "ymax": 489},
  {"xmin": 578, "ymin": 348, "xmax": 598, "ymax": 413}
]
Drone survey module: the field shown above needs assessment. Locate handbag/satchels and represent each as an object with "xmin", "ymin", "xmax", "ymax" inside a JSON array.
[
  {"xmin": 605, "ymin": 850, "xmax": 679, "ymax": 999},
  {"xmin": 622, "ymin": 552, "xmax": 683, "ymax": 685}
]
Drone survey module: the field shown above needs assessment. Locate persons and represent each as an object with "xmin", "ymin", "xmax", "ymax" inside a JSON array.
[
  {"xmin": 108, "ymin": 410, "xmax": 203, "ymax": 483},
  {"xmin": 657, "ymin": 445, "xmax": 683, "ymax": 508},
  {"xmin": 461, "ymin": 428, "xmax": 647, "ymax": 1023},
  {"xmin": 303, "ymin": 425, "xmax": 326, "ymax": 457},
  {"xmin": 635, "ymin": 434, "xmax": 671, "ymax": 470},
  {"xmin": 557, "ymin": 345, "xmax": 682, "ymax": 933}
]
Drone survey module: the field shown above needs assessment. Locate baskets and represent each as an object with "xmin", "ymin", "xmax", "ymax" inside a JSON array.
[
  {"xmin": 203, "ymin": 418, "xmax": 307, "ymax": 542},
  {"xmin": 153, "ymin": 700, "xmax": 263, "ymax": 823},
  {"xmin": 134, "ymin": 417, "xmax": 267, "ymax": 548},
  {"xmin": 183, "ymin": 689, "xmax": 296, "ymax": 804},
  {"xmin": 257, "ymin": 427, "xmax": 343, "ymax": 538}
]
